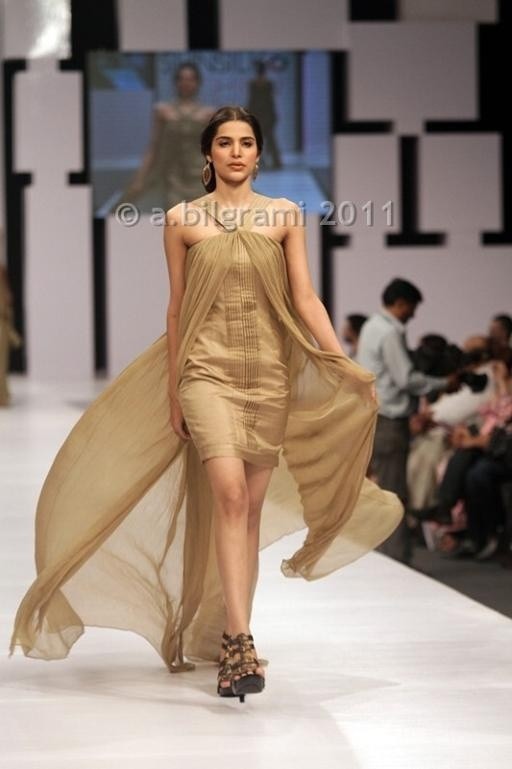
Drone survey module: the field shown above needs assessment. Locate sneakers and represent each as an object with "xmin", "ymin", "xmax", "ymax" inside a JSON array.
[
  {"xmin": 474, "ymin": 535, "xmax": 500, "ymax": 563},
  {"xmin": 420, "ymin": 519, "xmax": 437, "ymax": 553}
]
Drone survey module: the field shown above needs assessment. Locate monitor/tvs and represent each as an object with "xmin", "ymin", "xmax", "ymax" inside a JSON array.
[{"xmin": 81, "ymin": 47, "xmax": 337, "ymax": 221}]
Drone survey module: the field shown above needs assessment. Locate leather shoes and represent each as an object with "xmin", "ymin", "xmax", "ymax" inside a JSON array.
[{"xmin": 405, "ymin": 502, "xmax": 453, "ymax": 525}]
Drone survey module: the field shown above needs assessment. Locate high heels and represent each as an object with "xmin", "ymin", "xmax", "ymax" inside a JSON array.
[{"xmin": 217, "ymin": 629, "xmax": 266, "ymax": 704}]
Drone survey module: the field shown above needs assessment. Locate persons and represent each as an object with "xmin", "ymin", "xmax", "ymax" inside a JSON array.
[
  {"xmin": 342, "ymin": 277, "xmax": 511, "ymax": 568},
  {"xmin": 247, "ymin": 59, "xmax": 284, "ymax": 169},
  {"xmin": 163, "ymin": 107, "xmax": 346, "ymax": 701},
  {"xmin": 124, "ymin": 60, "xmax": 215, "ymax": 218}
]
